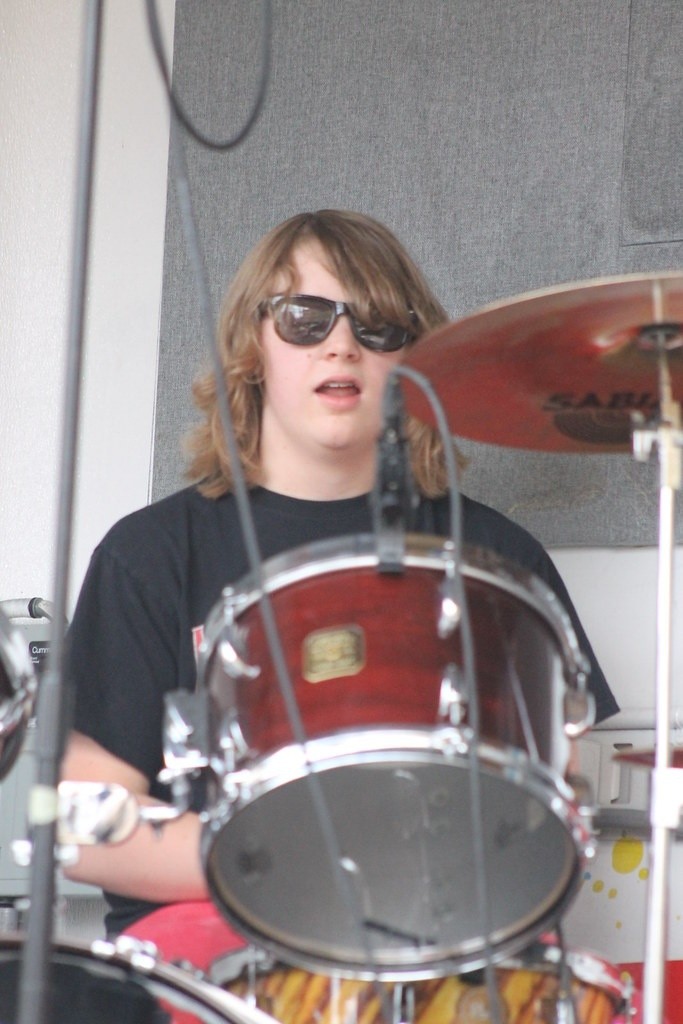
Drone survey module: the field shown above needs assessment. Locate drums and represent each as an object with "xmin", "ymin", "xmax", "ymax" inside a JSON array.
[{"xmin": 171, "ymin": 529, "xmax": 593, "ymax": 983}]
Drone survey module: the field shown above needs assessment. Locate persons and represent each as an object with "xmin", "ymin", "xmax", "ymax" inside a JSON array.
[{"xmin": 56, "ymin": 209, "xmax": 632, "ymax": 1023}]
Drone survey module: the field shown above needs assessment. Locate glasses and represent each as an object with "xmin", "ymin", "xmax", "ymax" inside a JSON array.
[{"xmin": 255, "ymin": 291, "xmax": 420, "ymax": 349}]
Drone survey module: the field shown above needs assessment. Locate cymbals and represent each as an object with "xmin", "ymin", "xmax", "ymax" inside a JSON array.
[{"xmin": 403, "ymin": 263, "xmax": 680, "ymax": 461}]
